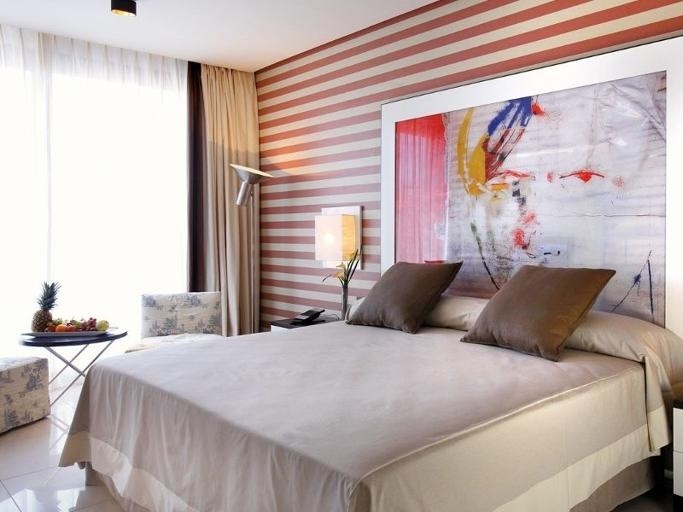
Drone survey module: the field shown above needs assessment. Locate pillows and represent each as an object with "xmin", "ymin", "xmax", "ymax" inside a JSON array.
[
  {"xmin": 457, "ymin": 264, "xmax": 618, "ymax": 363},
  {"xmin": 344, "ymin": 251, "xmax": 464, "ymax": 335}
]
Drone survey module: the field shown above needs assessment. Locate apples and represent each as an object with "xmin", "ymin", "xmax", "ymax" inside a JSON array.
[{"xmin": 44, "ymin": 326, "xmax": 55, "ymax": 332}]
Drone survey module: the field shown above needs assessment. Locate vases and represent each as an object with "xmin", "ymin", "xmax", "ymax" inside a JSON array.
[{"xmin": 339, "ymin": 284, "xmax": 349, "ymax": 321}]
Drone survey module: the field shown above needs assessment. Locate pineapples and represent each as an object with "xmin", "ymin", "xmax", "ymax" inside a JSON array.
[{"xmin": 31, "ymin": 310, "xmax": 52, "ymax": 333}]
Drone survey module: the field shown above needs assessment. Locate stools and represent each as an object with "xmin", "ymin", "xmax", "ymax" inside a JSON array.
[{"xmin": 0, "ymin": 354, "xmax": 51, "ymax": 437}]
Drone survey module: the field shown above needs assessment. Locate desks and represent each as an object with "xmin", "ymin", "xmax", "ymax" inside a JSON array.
[{"xmin": 18, "ymin": 326, "xmax": 129, "ymax": 408}]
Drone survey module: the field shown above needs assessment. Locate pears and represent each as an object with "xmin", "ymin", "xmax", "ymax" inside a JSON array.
[{"xmin": 96, "ymin": 320, "xmax": 109, "ymax": 331}]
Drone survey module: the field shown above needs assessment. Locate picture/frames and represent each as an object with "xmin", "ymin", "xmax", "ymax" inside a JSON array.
[{"xmin": 381, "ymin": 34, "xmax": 682, "ymax": 340}]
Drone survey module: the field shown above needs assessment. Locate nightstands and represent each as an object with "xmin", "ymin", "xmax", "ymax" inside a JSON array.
[
  {"xmin": 268, "ymin": 314, "xmax": 339, "ymax": 331},
  {"xmin": 668, "ymin": 393, "xmax": 682, "ymax": 498}
]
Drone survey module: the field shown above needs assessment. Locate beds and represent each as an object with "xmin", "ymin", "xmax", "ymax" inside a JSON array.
[{"xmin": 56, "ymin": 296, "xmax": 682, "ymax": 512}]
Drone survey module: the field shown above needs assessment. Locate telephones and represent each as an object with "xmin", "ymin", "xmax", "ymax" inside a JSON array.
[{"xmin": 291, "ymin": 309, "xmax": 325, "ymax": 324}]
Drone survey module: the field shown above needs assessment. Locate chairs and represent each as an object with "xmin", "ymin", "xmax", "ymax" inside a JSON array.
[{"xmin": 135, "ymin": 291, "xmax": 224, "ymax": 347}]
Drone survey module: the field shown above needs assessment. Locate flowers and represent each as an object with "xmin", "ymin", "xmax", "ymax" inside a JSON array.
[{"xmin": 321, "ymin": 249, "xmax": 361, "ymax": 314}]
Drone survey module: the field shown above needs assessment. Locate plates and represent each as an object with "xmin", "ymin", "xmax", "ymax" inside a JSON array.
[{"xmin": 19, "ymin": 329, "xmax": 106, "ymax": 338}]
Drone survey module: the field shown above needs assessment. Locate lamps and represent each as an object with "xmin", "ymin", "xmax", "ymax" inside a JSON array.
[
  {"xmin": 110, "ymin": 0, "xmax": 136, "ymax": 17},
  {"xmin": 309, "ymin": 203, "xmax": 365, "ymax": 271},
  {"xmin": 229, "ymin": 160, "xmax": 274, "ymax": 333}
]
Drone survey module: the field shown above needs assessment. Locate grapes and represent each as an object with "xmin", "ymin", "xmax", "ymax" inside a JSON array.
[{"xmin": 76, "ymin": 318, "xmax": 96, "ymax": 331}]
